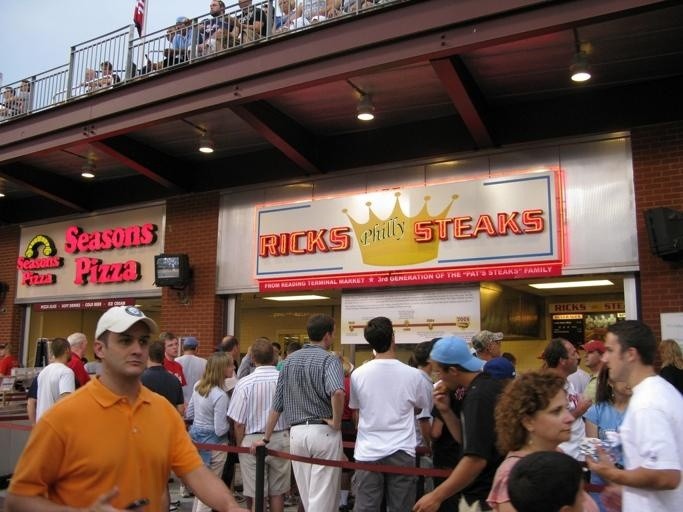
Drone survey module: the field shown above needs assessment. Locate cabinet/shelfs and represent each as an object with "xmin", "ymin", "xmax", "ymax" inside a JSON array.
[{"xmin": 0, "ymin": 366, "xmax": 43, "ymax": 479}]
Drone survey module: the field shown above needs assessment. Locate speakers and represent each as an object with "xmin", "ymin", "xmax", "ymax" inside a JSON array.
[{"xmin": 643, "ymin": 207, "xmax": 683, "ymax": 257}]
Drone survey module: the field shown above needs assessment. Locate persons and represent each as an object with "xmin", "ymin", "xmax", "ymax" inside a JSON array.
[
  {"xmin": 141, "ymin": 332, "xmax": 302, "ymax": 512},
  {"xmin": 132, "ymin": 0, "xmax": 386, "ymax": 78},
  {"xmin": 4, "ymin": 303, "xmax": 249, "ymax": 512},
  {"xmin": 2, "ymin": 343, "xmax": 19, "ymax": 374},
  {"xmin": 585, "ymin": 321, "xmax": 682, "ymax": 512},
  {"xmin": 80, "ymin": 61, "xmax": 119, "ymax": 94},
  {"xmin": 0, "ymin": 80, "xmax": 30, "ymax": 122},
  {"xmin": 413, "ymin": 329, "xmax": 682, "ymax": 512},
  {"xmin": 345, "ymin": 316, "xmax": 435, "ymax": 510},
  {"xmin": 27, "ymin": 333, "xmax": 91, "ymax": 426},
  {"xmin": 249, "ymin": 312, "xmax": 347, "ymax": 511}
]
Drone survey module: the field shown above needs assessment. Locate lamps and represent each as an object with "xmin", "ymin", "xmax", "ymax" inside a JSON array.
[
  {"xmin": 81, "ymin": 162, "xmax": 95, "ymax": 178},
  {"xmin": 354, "ymin": 92, "xmax": 376, "ymax": 122},
  {"xmin": 198, "ymin": 129, "xmax": 213, "ymax": 154},
  {"xmin": 569, "ymin": 50, "xmax": 591, "ymax": 84}
]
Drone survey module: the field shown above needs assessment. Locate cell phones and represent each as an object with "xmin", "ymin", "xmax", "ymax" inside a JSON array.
[{"xmin": 126, "ymin": 498, "xmax": 149, "ymax": 509}]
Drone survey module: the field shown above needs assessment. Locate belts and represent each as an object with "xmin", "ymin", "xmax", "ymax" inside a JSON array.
[{"xmin": 292, "ymin": 420, "xmax": 328, "ymax": 424}]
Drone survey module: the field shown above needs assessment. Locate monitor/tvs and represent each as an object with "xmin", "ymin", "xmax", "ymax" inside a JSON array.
[{"xmin": 155, "ymin": 255, "xmax": 190, "ymax": 285}]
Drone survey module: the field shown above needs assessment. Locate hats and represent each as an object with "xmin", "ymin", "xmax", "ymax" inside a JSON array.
[
  {"xmin": 185, "ymin": 337, "xmax": 199, "ymax": 345},
  {"xmin": 430, "ymin": 331, "xmax": 516, "ymax": 378},
  {"xmin": 580, "ymin": 340, "xmax": 605, "ymax": 353},
  {"xmin": 95, "ymin": 306, "xmax": 160, "ymax": 340}
]
